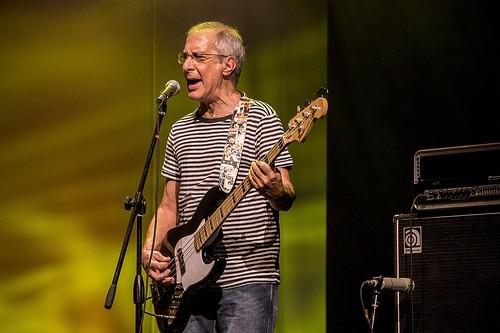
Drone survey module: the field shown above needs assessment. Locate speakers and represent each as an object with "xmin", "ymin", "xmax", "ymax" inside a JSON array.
[{"xmin": 392, "ymin": 207, "xmax": 500, "ymax": 333}]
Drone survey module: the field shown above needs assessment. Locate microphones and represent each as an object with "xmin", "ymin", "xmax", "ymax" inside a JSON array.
[
  {"xmin": 157, "ymin": 80, "xmax": 180, "ymax": 104},
  {"xmin": 369, "ymin": 277, "xmax": 415, "ymax": 293}
]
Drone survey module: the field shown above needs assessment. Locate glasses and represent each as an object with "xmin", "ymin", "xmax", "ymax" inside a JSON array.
[{"xmin": 177, "ymin": 52, "xmax": 229, "ymax": 64}]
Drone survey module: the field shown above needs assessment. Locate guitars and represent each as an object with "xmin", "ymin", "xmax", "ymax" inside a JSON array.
[{"xmin": 151, "ymin": 85, "xmax": 329, "ymax": 333}]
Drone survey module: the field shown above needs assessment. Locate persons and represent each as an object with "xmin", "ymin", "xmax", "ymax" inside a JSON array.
[{"xmin": 140, "ymin": 20, "xmax": 296, "ymax": 332}]
